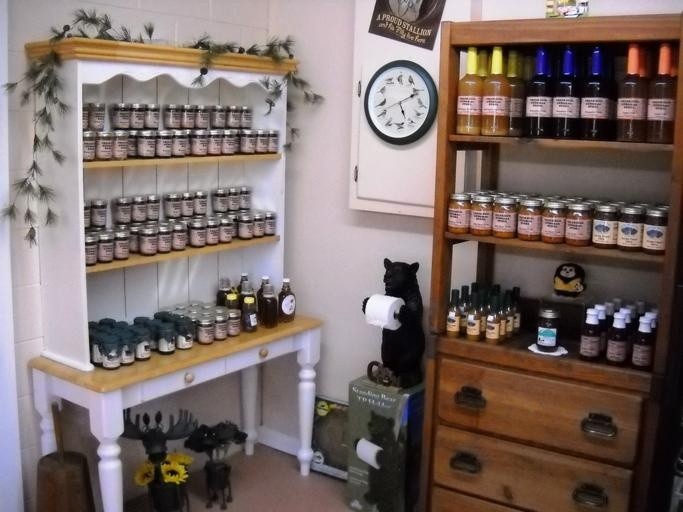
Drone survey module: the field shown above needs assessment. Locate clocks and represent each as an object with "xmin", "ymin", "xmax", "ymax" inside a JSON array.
[{"xmin": 362, "ymin": 60, "xmax": 439, "ymax": 146}]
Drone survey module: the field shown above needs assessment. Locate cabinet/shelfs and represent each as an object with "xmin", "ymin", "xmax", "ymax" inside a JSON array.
[
  {"xmin": 17, "ymin": 35, "xmax": 328, "ymax": 374},
  {"xmin": 416, "ymin": 11, "xmax": 683, "ymax": 506}
]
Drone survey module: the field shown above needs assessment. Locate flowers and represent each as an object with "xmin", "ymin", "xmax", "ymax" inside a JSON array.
[{"xmin": 133, "ymin": 451, "xmax": 194, "ymax": 486}]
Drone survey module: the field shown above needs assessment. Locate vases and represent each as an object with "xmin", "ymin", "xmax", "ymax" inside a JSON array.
[{"xmin": 146, "ymin": 482, "xmax": 184, "ymax": 511}]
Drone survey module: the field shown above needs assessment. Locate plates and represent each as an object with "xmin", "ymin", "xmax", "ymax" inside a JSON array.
[{"xmin": 528, "ymin": 343, "xmax": 568, "ymax": 357}]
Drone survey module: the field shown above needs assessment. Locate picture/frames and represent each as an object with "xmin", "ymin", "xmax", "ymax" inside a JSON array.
[{"xmin": 308, "ymin": 393, "xmax": 349, "ymax": 481}]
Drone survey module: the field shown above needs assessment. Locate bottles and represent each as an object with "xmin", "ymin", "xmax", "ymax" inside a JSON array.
[
  {"xmin": 216, "ymin": 273, "xmax": 296, "ymax": 333},
  {"xmin": 578, "ymin": 298, "xmax": 660, "ymax": 371},
  {"xmin": 457, "ymin": 43, "xmax": 679, "ymax": 145},
  {"xmin": 446, "ymin": 282, "xmax": 522, "ymax": 344}
]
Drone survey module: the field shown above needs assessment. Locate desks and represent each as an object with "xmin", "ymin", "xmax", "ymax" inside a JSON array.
[{"xmin": 26, "ymin": 311, "xmax": 327, "ymax": 511}]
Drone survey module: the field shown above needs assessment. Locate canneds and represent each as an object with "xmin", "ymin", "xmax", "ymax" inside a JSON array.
[
  {"xmin": 82, "ymin": 101, "xmax": 279, "ymax": 162},
  {"xmin": 83, "ymin": 185, "xmax": 276, "ymax": 266},
  {"xmin": 88, "ymin": 301, "xmax": 242, "ymax": 371},
  {"xmin": 447, "ymin": 190, "xmax": 670, "ymax": 256},
  {"xmin": 536, "ymin": 309, "xmax": 562, "ymax": 352}
]
